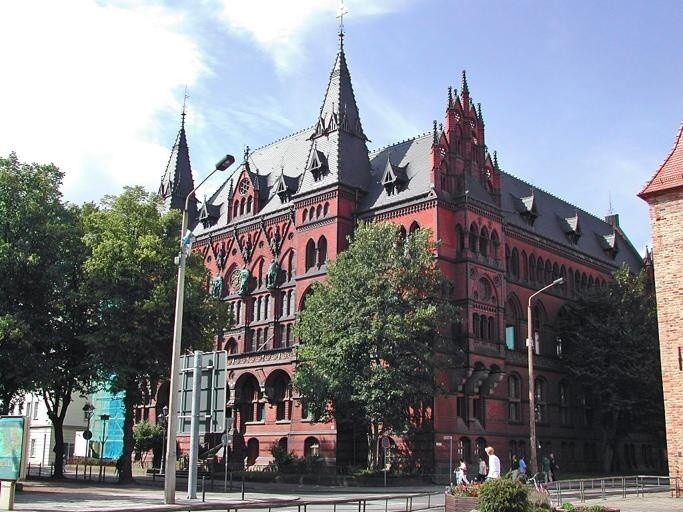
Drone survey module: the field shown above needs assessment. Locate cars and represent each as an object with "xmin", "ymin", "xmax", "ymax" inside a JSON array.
[{"xmin": 244, "ymin": 455, "xmax": 279, "ymax": 472}]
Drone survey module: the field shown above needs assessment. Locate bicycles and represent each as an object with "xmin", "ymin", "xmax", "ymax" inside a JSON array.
[{"xmin": 525, "ymin": 470, "xmax": 555, "ymax": 507}]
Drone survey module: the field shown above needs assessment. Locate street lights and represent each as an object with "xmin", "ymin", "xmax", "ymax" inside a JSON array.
[
  {"xmin": 162, "ymin": 151, "xmax": 238, "ymax": 509},
  {"xmin": 97, "ymin": 413, "xmax": 111, "ymax": 483},
  {"xmin": 82, "ymin": 402, "xmax": 95, "ymax": 481},
  {"xmin": 155, "ymin": 404, "xmax": 169, "ymax": 477},
  {"xmin": 525, "ymin": 276, "xmax": 568, "ymax": 479}
]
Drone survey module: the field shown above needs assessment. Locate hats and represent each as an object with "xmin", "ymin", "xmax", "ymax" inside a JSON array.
[{"xmin": 485, "ymin": 446, "xmax": 495, "ymax": 453}]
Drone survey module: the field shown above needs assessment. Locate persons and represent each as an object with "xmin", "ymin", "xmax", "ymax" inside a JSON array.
[
  {"xmin": 512, "ymin": 454, "xmax": 527, "ymax": 480},
  {"xmin": 453, "ymin": 459, "xmax": 469, "ymax": 486},
  {"xmin": 477, "ymin": 446, "xmax": 500, "ymax": 483},
  {"xmin": 542, "ymin": 453, "xmax": 559, "ymax": 483}
]
